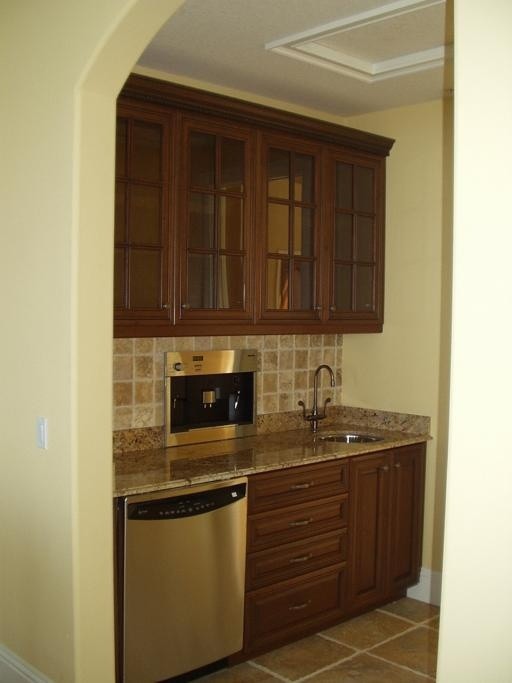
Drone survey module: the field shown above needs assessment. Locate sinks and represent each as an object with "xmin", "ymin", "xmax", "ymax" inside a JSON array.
[{"xmin": 318, "ymin": 431, "xmax": 382, "ymax": 444}]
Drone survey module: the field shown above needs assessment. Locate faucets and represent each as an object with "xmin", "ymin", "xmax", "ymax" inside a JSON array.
[{"xmin": 297, "ymin": 364, "xmax": 336, "ymax": 433}]
